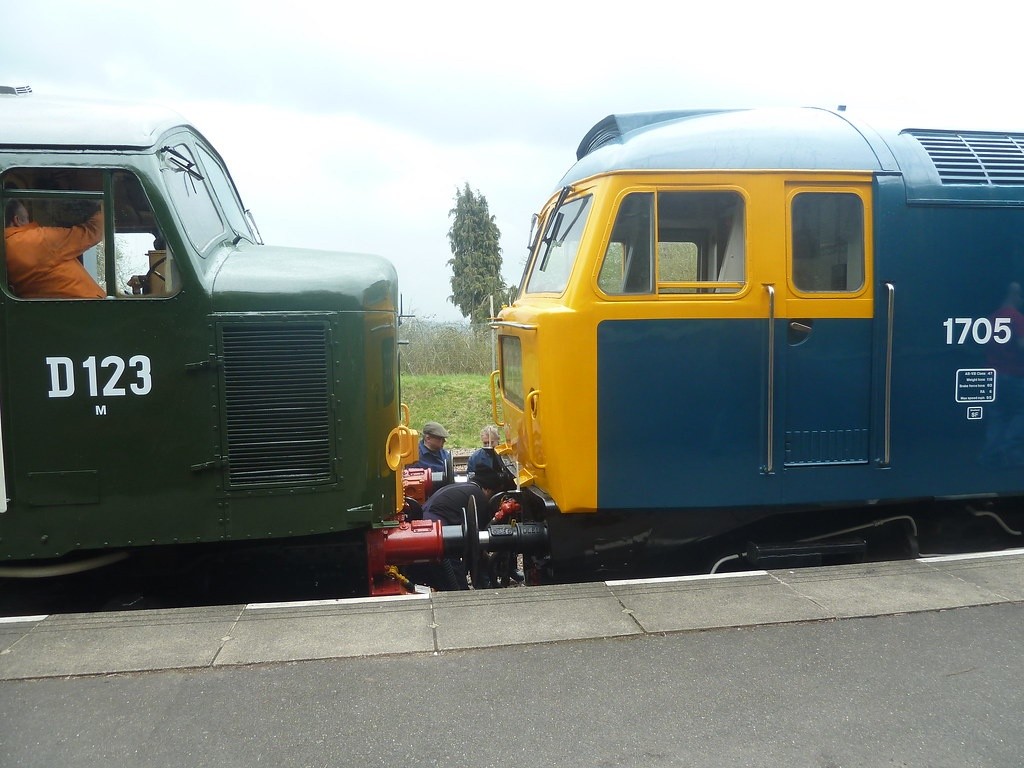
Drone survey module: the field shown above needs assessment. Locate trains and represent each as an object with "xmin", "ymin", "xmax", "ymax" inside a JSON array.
[{"xmin": 1, "ymin": 83, "xmax": 1024, "ymax": 611}]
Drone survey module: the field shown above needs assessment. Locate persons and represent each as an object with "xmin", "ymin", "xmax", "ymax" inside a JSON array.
[
  {"xmin": 4, "ymin": 199, "xmax": 106, "ymax": 299},
  {"xmin": 411, "ymin": 421, "xmax": 524, "ymax": 590}
]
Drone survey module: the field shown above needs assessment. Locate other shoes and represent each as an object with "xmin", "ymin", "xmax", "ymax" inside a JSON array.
[{"xmin": 512, "ymin": 571, "xmax": 524, "ymax": 582}]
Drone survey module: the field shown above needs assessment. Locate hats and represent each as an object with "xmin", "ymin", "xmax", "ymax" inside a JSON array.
[{"xmin": 423, "ymin": 422, "xmax": 449, "ymax": 438}]
